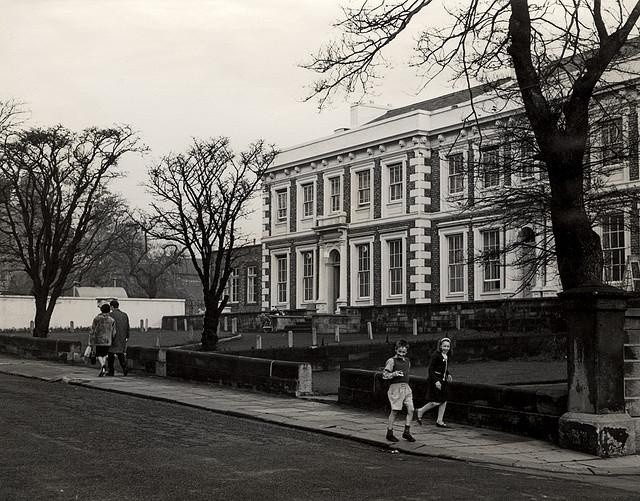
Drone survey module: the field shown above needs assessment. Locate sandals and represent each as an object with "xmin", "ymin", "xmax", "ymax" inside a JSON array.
[{"xmin": 414, "ymin": 408, "xmax": 451, "ymax": 429}]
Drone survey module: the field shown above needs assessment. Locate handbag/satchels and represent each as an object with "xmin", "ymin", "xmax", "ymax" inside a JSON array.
[{"xmin": 83, "ymin": 345, "xmax": 97, "ymax": 365}]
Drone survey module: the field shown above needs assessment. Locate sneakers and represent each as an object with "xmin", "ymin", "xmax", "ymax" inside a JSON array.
[
  {"xmin": 97, "ymin": 365, "xmax": 128, "ymax": 376},
  {"xmin": 386, "ymin": 433, "xmax": 416, "ymax": 443}
]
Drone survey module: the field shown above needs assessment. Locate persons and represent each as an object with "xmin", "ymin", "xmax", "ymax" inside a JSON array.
[
  {"xmin": 106, "ymin": 301, "xmax": 129, "ymax": 377},
  {"xmin": 381, "ymin": 336, "xmax": 418, "ymax": 444},
  {"xmin": 88, "ymin": 304, "xmax": 116, "ymax": 377},
  {"xmin": 415, "ymin": 337, "xmax": 455, "ymax": 428}
]
